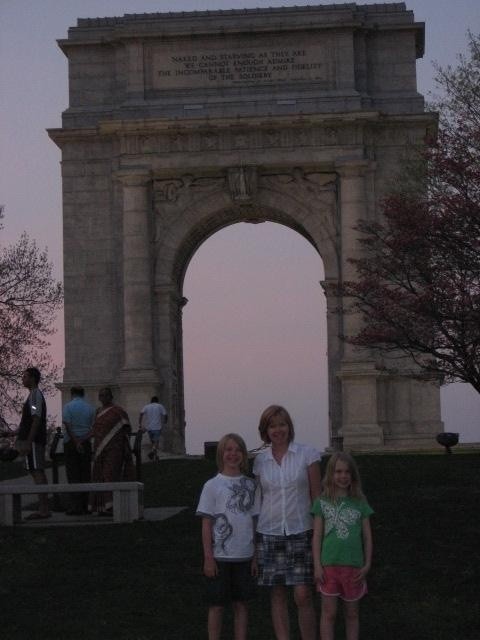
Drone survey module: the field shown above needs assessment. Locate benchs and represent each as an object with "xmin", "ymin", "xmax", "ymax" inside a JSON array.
[{"xmin": 0, "ymin": 481, "xmax": 144, "ymax": 527}]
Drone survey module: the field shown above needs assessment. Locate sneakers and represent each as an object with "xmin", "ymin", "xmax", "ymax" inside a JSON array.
[{"xmin": 67, "ymin": 506, "xmax": 88, "ymax": 515}]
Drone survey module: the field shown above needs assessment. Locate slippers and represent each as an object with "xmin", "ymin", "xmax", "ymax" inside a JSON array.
[{"xmin": 25, "ymin": 513, "xmax": 51, "ymax": 520}]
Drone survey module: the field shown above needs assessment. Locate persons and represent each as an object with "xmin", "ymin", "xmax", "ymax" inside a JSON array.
[
  {"xmin": 252, "ymin": 403, "xmax": 324, "ymax": 640},
  {"xmin": 53, "ymin": 426, "xmax": 63, "ymax": 447},
  {"xmin": 138, "ymin": 396, "xmax": 169, "ymax": 460},
  {"xmin": 308, "ymin": 450, "xmax": 374, "ymax": 640},
  {"xmin": 16, "ymin": 366, "xmax": 53, "ymax": 521},
  {"xmin": 61, "ymin": 382, "xmax": 97, "ymax": 516},
  {"xmin": 194, "ymin": 433, "xmax": 264, "ymax": 640},
  {"xmin": 87, "ymin": 387, "xmax": 132, "ymax": 518}
]
